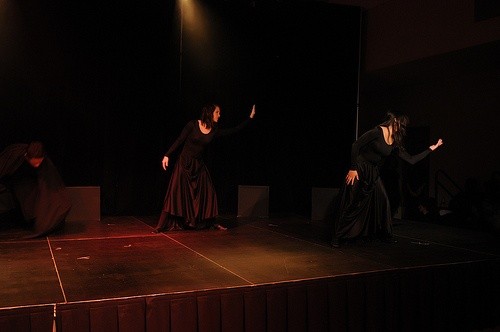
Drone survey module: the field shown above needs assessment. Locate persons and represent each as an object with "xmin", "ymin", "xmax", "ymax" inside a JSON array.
[
  {"xmin": 0, "ymin": 142, "xmax": 71, "ymax": 239},
  {"xmin": 330, "ymin": 109, "xmax": 443, "ymax": 249},
  {"xmin": 153, "ymin": 103, "xmax": 255, "ymax": 231},
  {"xmin": 418, "ymin": 197, "xmax": 440, "ymax": 224}
]
